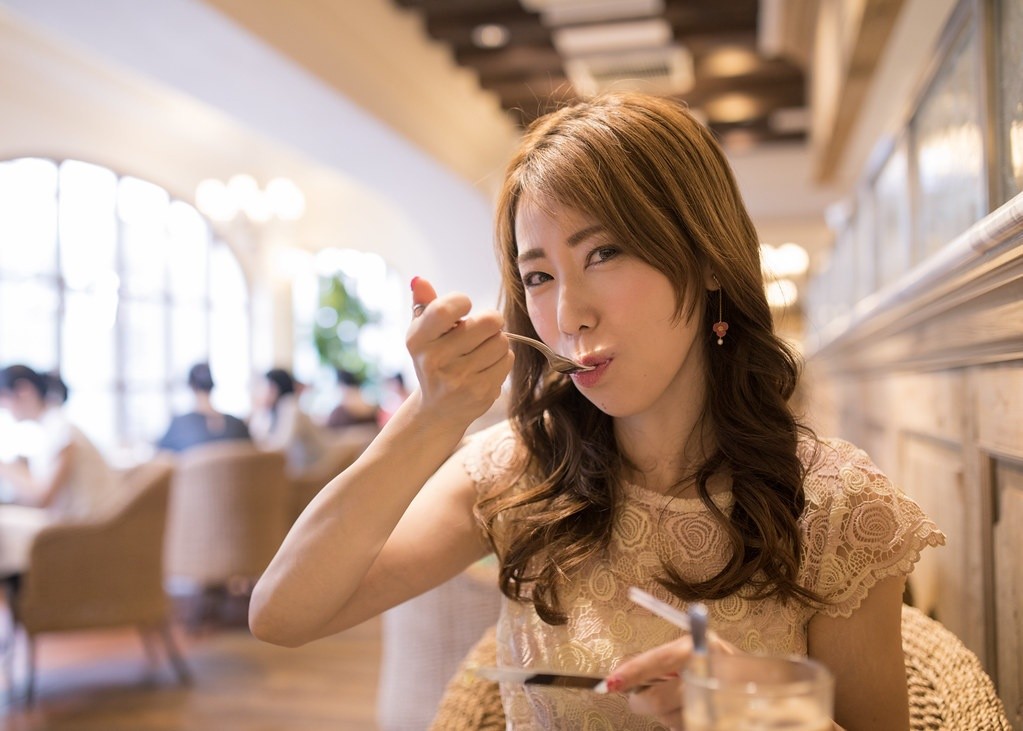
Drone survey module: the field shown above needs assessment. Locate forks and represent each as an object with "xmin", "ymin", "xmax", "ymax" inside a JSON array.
[{"xmin": 414, "ymin": 305, "xmax": 595, "ymax": 375}]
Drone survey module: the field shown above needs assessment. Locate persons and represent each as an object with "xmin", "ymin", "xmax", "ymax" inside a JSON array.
[
  {"xmin": 2, "ymin": 364, "xmax": 122, "ymax": 607},
  {"xmin": 249, "ymin": 93, "xmax": 947, "ymax": 731},
  {"xmin": 153, "ymin": 361, "xmax": 408, "ymax": 453}
]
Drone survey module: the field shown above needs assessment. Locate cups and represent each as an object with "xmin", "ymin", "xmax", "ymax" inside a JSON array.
[{"xmin": 677, "ymin": 653, "xmax": 836, "ymax": 731}]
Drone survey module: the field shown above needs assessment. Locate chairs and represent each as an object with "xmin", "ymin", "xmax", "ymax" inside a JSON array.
[
  {"xmin": 166, "ymin": 444, "xmax": 286, "ymax": 601},
  {"xmin": 0, "ymin": 456, "xmax": 197, "ymax": 712},
  {"xmin": 431, "ymin": 601, "xmax": 1015, "ymax": 731}
]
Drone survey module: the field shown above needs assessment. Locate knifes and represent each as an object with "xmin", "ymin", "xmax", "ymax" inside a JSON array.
[{"xmin": 481, "ymin": 667, "xmax": 654, "ymax": 694}]
